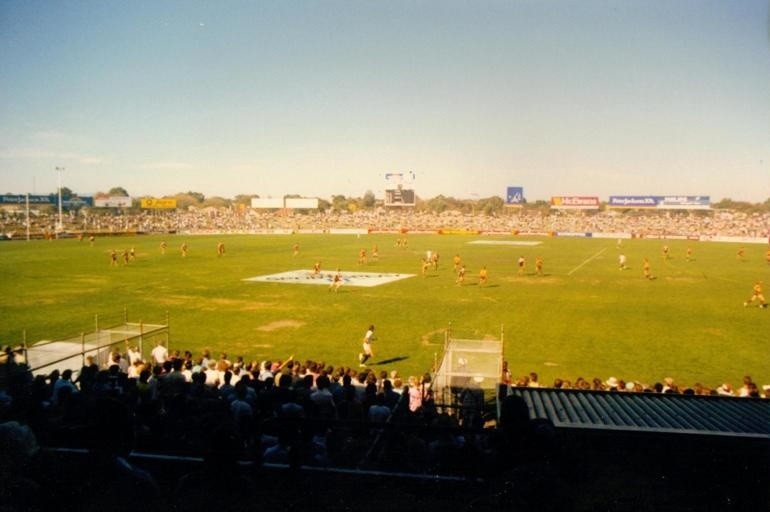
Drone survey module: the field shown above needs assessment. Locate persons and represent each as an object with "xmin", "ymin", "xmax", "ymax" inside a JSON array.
[
  {"xmin": 643, "ymin": 257, "xmax": 650, "ymax": 278},
  {"xmin": 517, "ymin": 255, "xmax": 526, "ymax": 275},
  {"xmin": 313, "ymin": 258, "xmax": 323, "ymax": 279},
  {"xmin": 0, "ymin": 337, "xmax": 769, "ymax": 512},
  {"xmin": 455, "ymin": 265, "xmax": 466, "ymax": 286},
  {"xmin": 535, "ymin": 255, "xmax": 545, "ymax": 277},
  {"xmin": 329, "ymin": 267, "xmax": 343, "ymax": 293},
  {"xmin": 0, "ymin": 205, "xmax": 770, "ymax": 274},
  {"xmin": 741, "ymin": 280, "xmax": 766, "ymax": 308},
  {"xmin": 477, "ymin": 266, "xmax": 487, "ymax": 285},
  {"xmin": 358, "ymin": 323, "xmax": 380, "ymax": 368}
]
